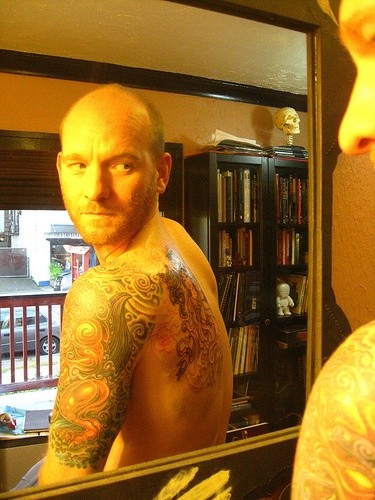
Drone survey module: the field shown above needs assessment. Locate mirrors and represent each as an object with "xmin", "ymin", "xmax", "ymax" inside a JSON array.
[{"xmin": 0, "ymin": 0, "xmax": 331, "ymax": 500}]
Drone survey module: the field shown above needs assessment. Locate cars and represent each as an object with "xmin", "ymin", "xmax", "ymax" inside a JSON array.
[{"xmin": 0, "ymin": 308, "xmax": 60, "ymax": 356}]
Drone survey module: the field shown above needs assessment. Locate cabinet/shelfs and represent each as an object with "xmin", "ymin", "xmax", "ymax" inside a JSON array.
[{"xmin": 180, "ymin": 150, "xmax": 307, "ymax": 440}]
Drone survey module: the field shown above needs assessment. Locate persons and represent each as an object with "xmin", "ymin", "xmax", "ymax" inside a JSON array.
[
  {"xmin": 37, "ymin": 83, "xmax": 232, "ymax": 487},
  {"xmin": 276, "ymin": 283, "xmax": 294, "ymax": 316},
  {"xmin": 53, "ymin": 276, "xmax": 64, "ymax": 291},
  {"xmin": 291, "ymin": 0, "xmax": 375, "ymax": 500}
]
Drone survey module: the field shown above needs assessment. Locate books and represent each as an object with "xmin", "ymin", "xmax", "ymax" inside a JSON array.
[
  {"xmin": 23, "ymin": 409, "xmax": 54, "ymax": 433},
  {"xmin": 216, "ymin": 166, "xmax": 306, "ymax": 431}
]
